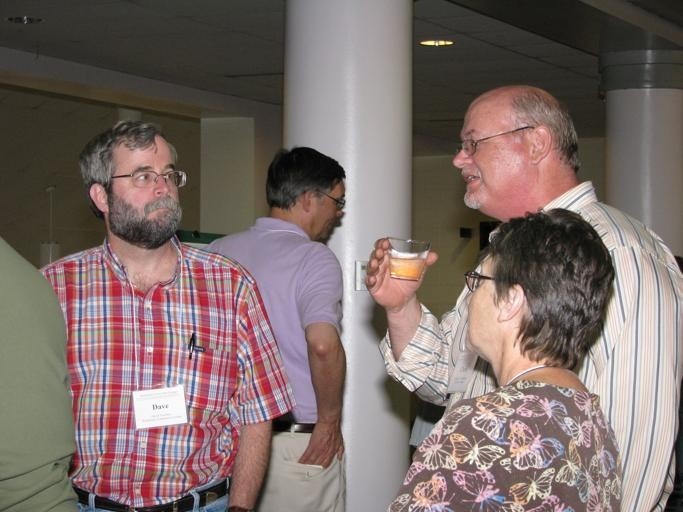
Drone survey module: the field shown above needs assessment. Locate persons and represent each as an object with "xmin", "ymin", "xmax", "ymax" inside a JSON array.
[
  {"xmin": 200, "ymin": 147, "xmax": 346, "ymax": 512},
  {"xmin": 39, "ymin": 119, "xmax": 297, "ymax": 512},
  {"xmin": 384, "ymin": 208, "xmax": 623, "ymax": 512},
  {"xmin": 366, "ymin": 84, "xmax": 683, "ymax": 512},
  {"xmin": 0, "ymin": 236, "xmax": 81, "ymax": 512}
]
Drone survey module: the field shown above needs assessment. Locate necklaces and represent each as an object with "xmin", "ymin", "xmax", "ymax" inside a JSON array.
[{"xmin": 504, "ymin": 361, "xmax": 553, "ymax": 383}]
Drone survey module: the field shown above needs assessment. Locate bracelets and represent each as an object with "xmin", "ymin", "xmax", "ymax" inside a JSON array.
[{"xmin": 225, "ymin": 506, "xmax": 256, "ymax": 512}]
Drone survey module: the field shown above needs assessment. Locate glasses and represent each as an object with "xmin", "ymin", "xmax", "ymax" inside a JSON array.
[
  {"xmin": 303, "ymin": 188, "xmax": 344, "ymax": 210},
  {"xmin": 463, "ymin": 270, "xmax": 495, "ymax": 293},
  {"xmin": 454, "ymin": 125, "xmax": 534, "ymax": 157},
  {"xmin": 110, "ymin": 170, "xmax": 187, "ymax": 190}
]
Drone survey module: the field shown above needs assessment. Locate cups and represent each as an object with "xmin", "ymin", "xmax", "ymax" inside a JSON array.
[{"xmin": 386, "ymin": 235, "xmax": 431, "ymax": 283}]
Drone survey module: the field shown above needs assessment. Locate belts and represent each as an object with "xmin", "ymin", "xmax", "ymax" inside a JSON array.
[
  {"xmin": 272, "ymin": 420, "xmax": 315, "ymax": 434},
  {"xmin": 73, "ymin": 479, "xmax": 230, "ymax": 512}
]
